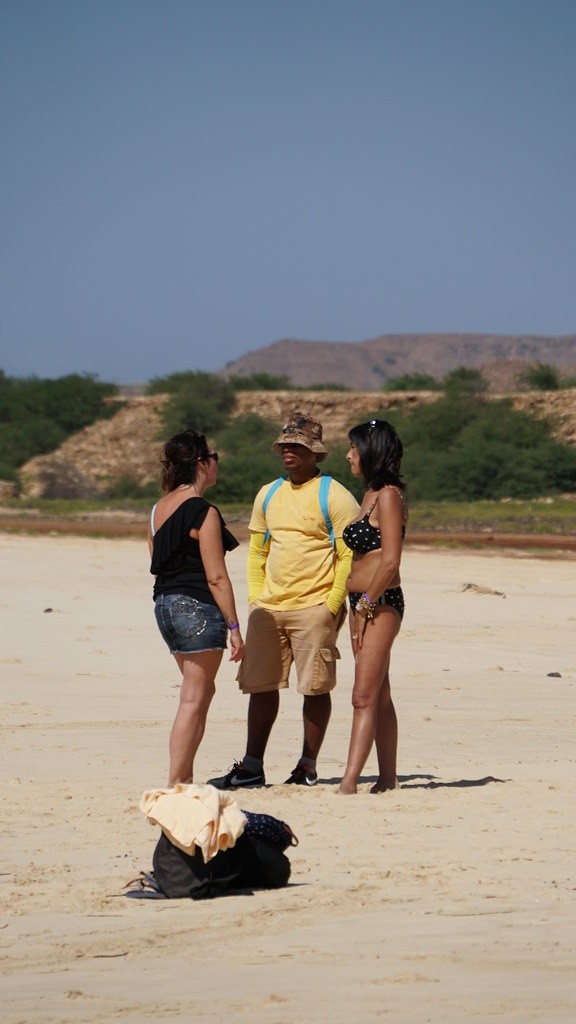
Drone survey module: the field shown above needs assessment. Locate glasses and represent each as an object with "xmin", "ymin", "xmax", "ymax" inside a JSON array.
[
  {"xmin": 206, "ymin": 453, "xmax": 218, "ymax": 463},
  {"xmin": 368, "ymin": 419, "xmax": 379, "ymax": 439}
]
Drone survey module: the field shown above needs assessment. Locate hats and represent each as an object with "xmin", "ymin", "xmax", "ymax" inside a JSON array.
[{"xmin": 273, "ymin": 411, "xmax": 328, "ymax": 463}]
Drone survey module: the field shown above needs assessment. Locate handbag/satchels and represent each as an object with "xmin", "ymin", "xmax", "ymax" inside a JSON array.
[{"xmin": 125, "ymin": 809, "xmax": 299, "ymax": 899}]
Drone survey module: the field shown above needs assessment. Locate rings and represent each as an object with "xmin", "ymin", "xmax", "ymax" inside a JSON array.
[{"xmin": 352, "ymin": 636, "xmax": 357, "ymax": 639}]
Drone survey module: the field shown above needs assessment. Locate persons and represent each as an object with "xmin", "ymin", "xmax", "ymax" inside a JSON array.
[
  {"xmin": 147, "ymin": 429, "xmax": 245, "ymax": 789},
  {"xmin": 202, "ymin": 413, "xmax": 361, "ymax": 789},
  {"xmin": 336, "ymin": 419, "xmax": 409, "ymax": 796}
]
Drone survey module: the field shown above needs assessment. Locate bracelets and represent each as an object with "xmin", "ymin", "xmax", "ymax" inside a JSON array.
[
  {"xmin": 229, "ymin": 623, "xmax": 239, "ymax": 630},
  {"xmin": 355, "ymin": 594, "xmax": 376, "ymax": 618}
]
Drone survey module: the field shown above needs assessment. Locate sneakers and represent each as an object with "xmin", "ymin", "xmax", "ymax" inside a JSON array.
[
  {"xmin": 206, "ymin": 758, "xmax": 266, "ymax": 789},
  {"xmin": 284, "ymin": 760, "xmax": 318, "ymax": 786}
]
